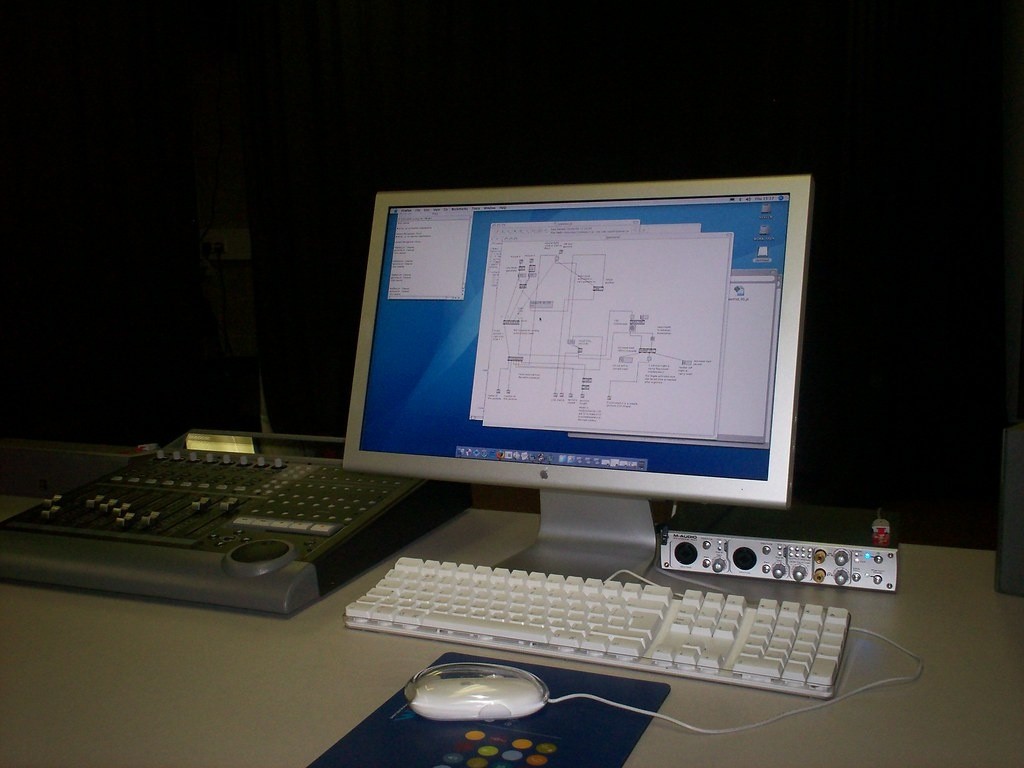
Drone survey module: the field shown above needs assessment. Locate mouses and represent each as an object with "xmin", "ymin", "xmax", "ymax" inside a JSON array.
[{"xmin": 405, "ymin": 663, "xmax": 550, "ymax": 719}]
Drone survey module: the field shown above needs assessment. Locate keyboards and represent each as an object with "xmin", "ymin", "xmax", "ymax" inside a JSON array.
[{"xmin": 343, "ymin": 559, "xmax": 851, "ymax": 699}]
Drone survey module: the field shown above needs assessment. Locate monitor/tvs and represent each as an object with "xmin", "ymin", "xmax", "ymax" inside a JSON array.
[{"xmin": 345, "ymin": 175, "xmax": 815, "ymax": 585}]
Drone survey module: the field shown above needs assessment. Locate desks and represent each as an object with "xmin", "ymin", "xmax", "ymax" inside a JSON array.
[{"xmin": 0, "ymin": 495, "xmax": 1024, "ymax": 768}]
